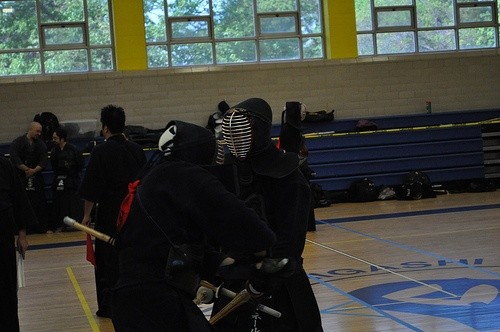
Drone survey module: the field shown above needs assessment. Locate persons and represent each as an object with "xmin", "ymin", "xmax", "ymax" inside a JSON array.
[{"xmin": 0, "ymin": 98, "xmax": 325, "ymax": 332}]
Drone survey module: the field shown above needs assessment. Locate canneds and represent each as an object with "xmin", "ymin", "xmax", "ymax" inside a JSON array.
[{"xmin": 425, "ymin": 101, "xmax": 431, "ymax": 114}]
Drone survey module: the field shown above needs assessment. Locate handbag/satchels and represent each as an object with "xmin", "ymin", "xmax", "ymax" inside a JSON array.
[{"xmin": 349, "ymin": 178, "xmax": 375, "ymax": 201}]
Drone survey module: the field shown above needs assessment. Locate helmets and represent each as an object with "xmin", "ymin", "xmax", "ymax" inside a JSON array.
[
  {"xmin": 158, "ymin": 120, "xmax": 213, "ymax": 171},
  {"xmin": 221, "ymin": 98, "xmax": 273, "ymax": 161}
]
